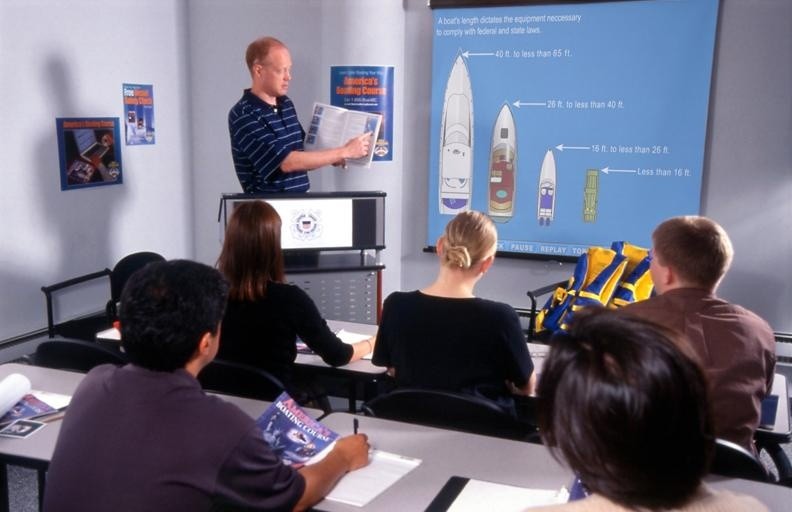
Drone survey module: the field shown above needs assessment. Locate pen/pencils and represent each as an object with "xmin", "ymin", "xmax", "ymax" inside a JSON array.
[
  {"xmin": 42, "ymin": 415, "xmax": 66, "ymax": 423},
  {"xmin": 354, "ymin": 419, "xmax": 358, "ymax": 435},
  {"xmin": 577, "ymin": 479, "xmax": 589, "ymax": 498}
]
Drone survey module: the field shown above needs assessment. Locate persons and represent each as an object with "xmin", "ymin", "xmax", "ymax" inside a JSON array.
[
  {"xmin": 521, "ymin": 306, "xmax": 772, "ymax": 512},
  {"xmin": 41, "ymin": 258, "xmax": 370, "ymax": 512},
  {"xmin": 370, "ymin": 209, "xmax": 537, "ymax": 431},
  {"xmin": 227, "ymin": 36, "xmax": 373, "ymax": 194},
  {"xmin": 91, "ymin": 133, "xmax": 117, "ymax": 181},
  {"xmin": 197, "ymin": 201, "xmax": 377, "ymax": 407},
  {"xmin": 621, "ymin": 216, "xmax": 778, "ymax": 477}
]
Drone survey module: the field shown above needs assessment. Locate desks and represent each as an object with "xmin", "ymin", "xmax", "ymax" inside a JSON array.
[
  {"xmin": 2, "ymin": 358, "xmax": 792, "ymax": 512},
  {"xmin": 95, "ymin": 315, "xmax": 791, "ymax": 453}
]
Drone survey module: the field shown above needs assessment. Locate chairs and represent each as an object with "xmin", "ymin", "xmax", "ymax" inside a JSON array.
[{"xmin": 30, "ymin": 340, "xmax": 769, "ymax": 483}]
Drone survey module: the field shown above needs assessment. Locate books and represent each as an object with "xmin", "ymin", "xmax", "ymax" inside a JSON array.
[
  {"xmin": 296, "ymin": 326, "xmax": 374, "ymax": 360},
  {"xmin": 303, "ymin": 102, "xmax": 383, "ymax": 165},
  {"xmin": 0, "ymin": 373, "xmax": 73, "ymax": 432},
  {"xmin": 252, "ymin": 391, "xmax": 423, "ymax": 508}
]
